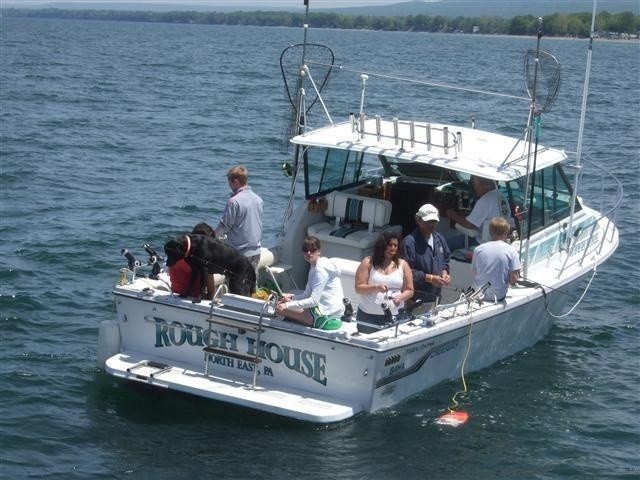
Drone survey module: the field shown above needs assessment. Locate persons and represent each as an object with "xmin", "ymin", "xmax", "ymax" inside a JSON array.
[
  {"xmin": 214, "ymin": 166, "xmax": 265, "ymax": 290},
  {"xmin": 445, "ymin": 175, "xmax": 512, "ymax": 261},
  {"xmin": 470, "ymin": 216, "xmax": 522, "ymax": 302},
  {"xmin": 167, "ymin": 222, "xmax": 226, "ymax": 297},
  {"xmin": 274, "ymin": 236, "xmax": 346, "ymax": 330},
  {"xmin": 397, "ymin": 203, "xmax": 453, "ymax": 316},
  {"xmin": 354, "ymin": 228, "xmax": 415, "ymax": 334}
]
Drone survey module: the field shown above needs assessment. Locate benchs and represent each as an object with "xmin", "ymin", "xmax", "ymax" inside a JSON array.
[{"xmin": 308, "ymin": 191, "xmax": 403, "ymax": 260}]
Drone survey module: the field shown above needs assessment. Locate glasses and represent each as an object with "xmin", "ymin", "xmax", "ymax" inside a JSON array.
[{"xmin": 303, "ymin": 247, "xmax": 318, "ymax": 253}]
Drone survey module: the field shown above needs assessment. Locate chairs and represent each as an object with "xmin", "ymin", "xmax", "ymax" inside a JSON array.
[{"xmin": 255, "ymin": 248, "xmax": 299, "ymax": 294}]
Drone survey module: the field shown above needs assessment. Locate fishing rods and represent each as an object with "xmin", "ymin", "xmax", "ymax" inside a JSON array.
[
  {"xmin": 519, "ymin": 276, "xmax": 612, "ymax": 316},
  {"xmin": 134, "ymin": 238, "xmax": 291, "ymax": 303},
  {"xmin": 282, "ymin": 0, "xmax": 308, "ymax": 194},
  {"xmin": 566, "ymin": 0, "xmax": 597, "ymax": 253}
]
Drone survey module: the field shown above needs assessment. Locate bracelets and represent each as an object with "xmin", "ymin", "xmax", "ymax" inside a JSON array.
[
  {"xmin": 430, "ymin": 274, "xmax": 433, "ymax": 283},
  {"xmin": 375, "ymin": 284, "xmax": 378, "ymax": 290}
]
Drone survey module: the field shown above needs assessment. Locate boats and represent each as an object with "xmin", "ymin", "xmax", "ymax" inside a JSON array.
[{"xmin": 93, "ymin": 3, "xmax": 625, "ymax": 431}]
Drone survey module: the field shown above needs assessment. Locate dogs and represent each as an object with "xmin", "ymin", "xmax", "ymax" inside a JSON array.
[{"xmin": 164, "ymin": 233, "xmax": 256, "ymax": 303}]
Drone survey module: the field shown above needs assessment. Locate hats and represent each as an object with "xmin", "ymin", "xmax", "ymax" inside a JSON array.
[
  {"xmin": 164, "ymin": 240, "xmax": 181, "ymax": 266},
  {"xmin": 416, "ymin": 204, "xmax": 440, "ymax": 222}
]
